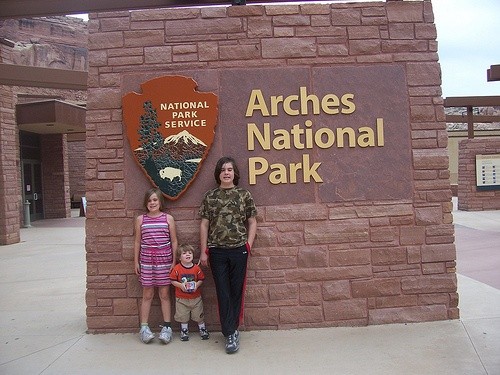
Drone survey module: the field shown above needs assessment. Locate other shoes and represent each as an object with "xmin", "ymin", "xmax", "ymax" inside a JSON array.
[
  {"xmin": 225, "ymin": 329, "xmax": 240, "ymax": 352},
  {"xmin": 139, "ymin": 328, "xmax": 155, "ymax": 344},
  {"xmin": 158, "ymin": 325, "xmax": 173, "ymax": 344},
  {"xmin": 200, "ymin": 327, "xmax": 209, "ymax": 340},
  {"xmin": 180, "ymin": 328, "xmax": 190, "ymax": 341}
]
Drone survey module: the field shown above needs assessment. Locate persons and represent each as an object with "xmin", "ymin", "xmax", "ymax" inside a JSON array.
[
  {"xmin": 169, "ymin": 244, "xmax": 209, "ymax": 342},
  {"xmin": 197, "ymin": 157, "xmax": 258, "ymax": 354},
  {"xmin": 133, "ymin": 187, "xmax": 178, "ymax": 345}
]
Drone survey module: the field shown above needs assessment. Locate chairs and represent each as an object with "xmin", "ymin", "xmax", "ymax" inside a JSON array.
[{"xmin": 72, "ymin": 194, "xmax": 83, "ymax": 216}]
